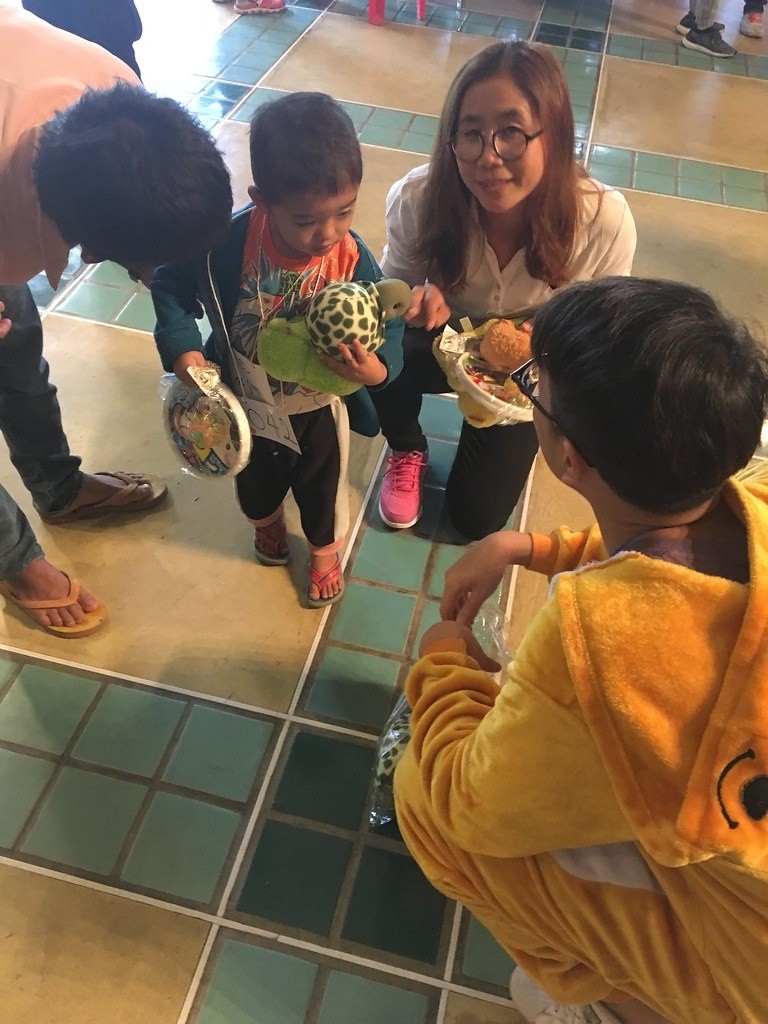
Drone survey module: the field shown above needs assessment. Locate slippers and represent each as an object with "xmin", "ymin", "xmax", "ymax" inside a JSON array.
[
  {"xmin": 304, "ymin": 550, "xmax": 345, "ymax": 610},
  {"xmin": 39, "ymin": 465, "xmax": 169, "ymax": 526},
  {"xmin": 1, "ymin": 562, "xmax": 110, "ymax": 640},
  {"xmin": 253, "ymin": 522, "xmax": 292, "ymax": 567}
]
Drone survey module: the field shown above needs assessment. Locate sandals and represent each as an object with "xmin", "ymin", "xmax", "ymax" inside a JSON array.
[{"xmin": 235, "ymin": 0, "xmax": 288, "ymax": 15}]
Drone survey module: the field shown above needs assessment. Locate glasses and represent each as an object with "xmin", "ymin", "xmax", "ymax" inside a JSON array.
[
  {"xmin": 446, "ymin": 119, "xmax": 544, "ymax": 163},
  {"xmin": 507, "ymin": 350, "xmax": 594, "ymax": 473}
]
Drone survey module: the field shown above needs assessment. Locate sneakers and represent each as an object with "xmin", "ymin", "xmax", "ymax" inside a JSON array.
[
  {"xmin": 681, "ymin": 22, "xmax": 738, "ymax": 59},
  {"xmin": 739, "ymin": 10, "xmax": 764, "ymax": 39},
  {"xmin": 507, "ymin": 964, "xmax": 624, "ymax": 1024},
  {"xmin": 677, "ymin": 11, "xmax": 725, "ymax": 39},
  {"xmin": 378, "ymin": 447, "xmax": 431, "ymax": 531}
]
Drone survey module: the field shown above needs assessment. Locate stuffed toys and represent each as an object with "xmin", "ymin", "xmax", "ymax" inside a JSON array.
[{"xmin": 256, "ymin": 280, "xmax": 411, "ymax": 398}]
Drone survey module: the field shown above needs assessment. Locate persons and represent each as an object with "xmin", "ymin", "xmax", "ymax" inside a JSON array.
[
  {"xmin": 0, "ymin": 5, "xmax": 234, "ymax": 638},
  {"xmin": 675, "ymin": 0, "xmax": 737, "ymax": 57},
  {"xmin": 391, "ymin": 276, "xmax": 768, "ymax": 1024},
  {"xmin": 740, "ymin": 0, "xmax": 768, "ymax": 37},
  {"xmin": 365, "ymin": 39, "xmax": 639, "ymax": 539},
  {"xmin": 212, "ymin": 0, "xmax": 286, "ymax": 14},
  {"xmin": 151, "ymin": 92, "xmax": 405, "ymax": 606}
]
